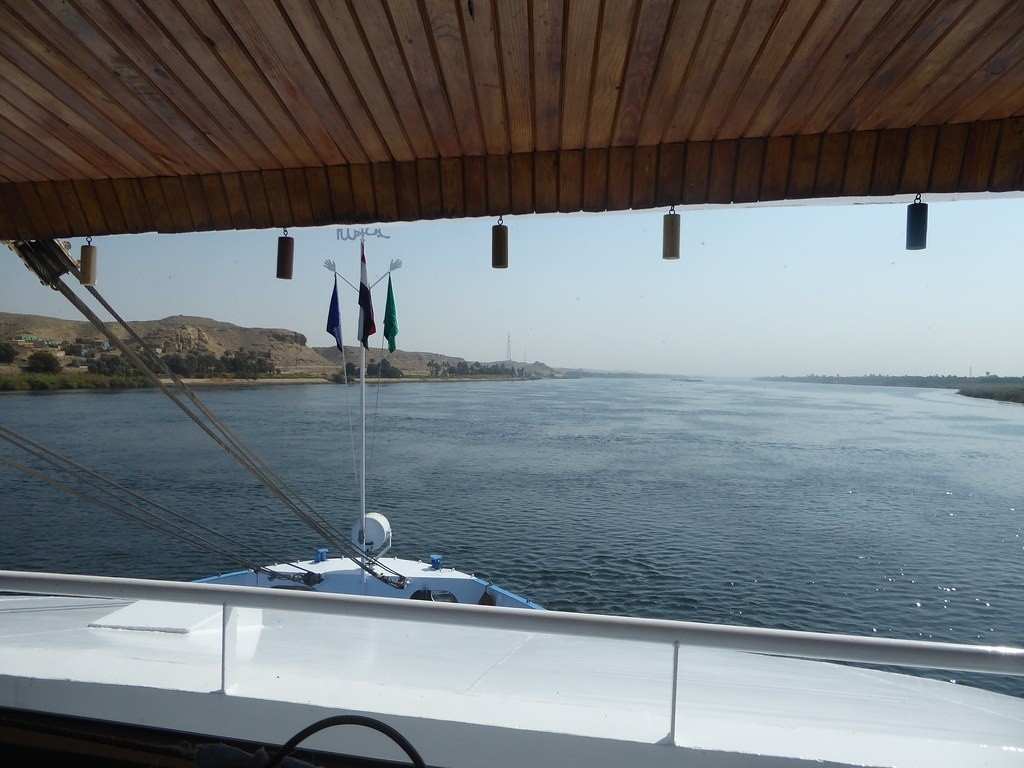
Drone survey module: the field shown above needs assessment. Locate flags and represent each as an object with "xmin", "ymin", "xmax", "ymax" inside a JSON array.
[
  {"xmin": 383, "ymin": 274, "xmax": 399, "ymax": 353},
  {"xmin": 326, "ymin": 281, "xmax": 343, "ymax": 352},
  {"xmin": 358, "ymin": 254, "xmax": 376, "ymax": 351}
]
